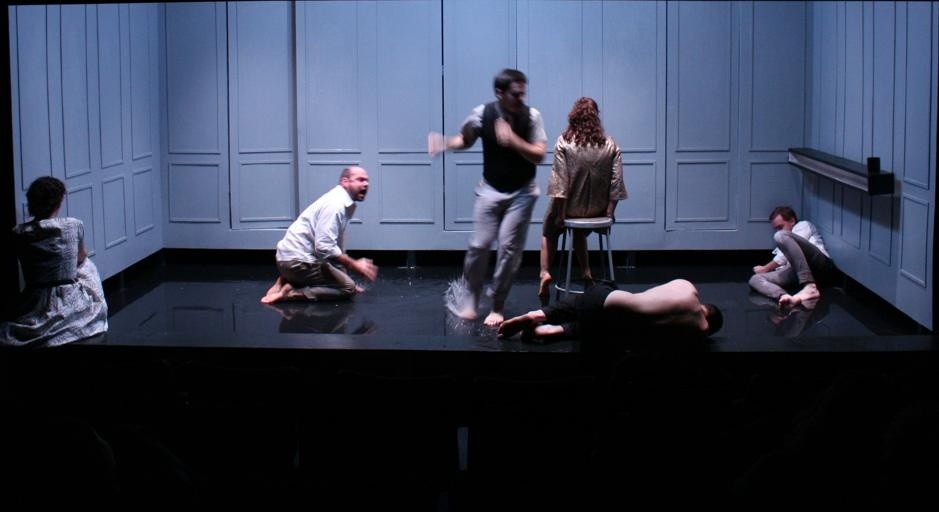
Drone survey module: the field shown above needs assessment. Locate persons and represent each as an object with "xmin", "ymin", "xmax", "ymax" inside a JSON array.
[
  {"xmin": 748, "ymin": 207, "xmax": 837, "ymax": 310},
  {"xmin": 263, "ymin": 302, "xmax": 376, "ymax": 335},
  {"xmin": 538, "ymin": 97, "xmax": 628, "ymax": 307},
  {"xmin": 0, "ymin": 176, "xmax": 109, "ymax": 353},
  {"xmin": 259, "ymin": 165, "xmax": 378, "ymax": 305},
  {"xmin": 426, "ymin": 68, "xmax": 548, "ymax": 327},
  {"xmin": 496, "ymin": 279, "xmax": 723, "ymax": 345},
  {"xmin": 749, "ymin": 289, "xmax": 835, "ymax": 338}
]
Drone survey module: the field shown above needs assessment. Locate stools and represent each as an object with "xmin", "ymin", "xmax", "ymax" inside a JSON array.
[{"xmin": 555, "ymin": 216, "xmax": 616, "ymax": 303}]
